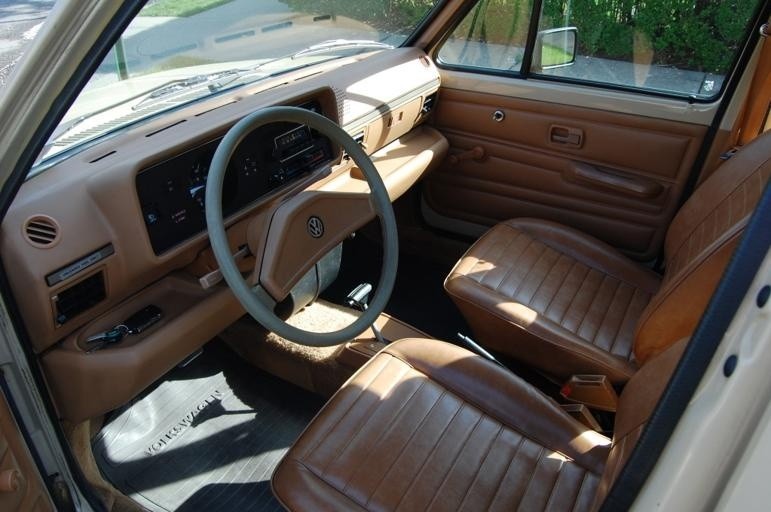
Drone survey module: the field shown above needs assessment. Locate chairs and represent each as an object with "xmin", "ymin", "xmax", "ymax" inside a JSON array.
[
  {"xmin": 270, "ymin": 338, "xmax": 698, "ymax": 512},
  {"xmin": 443, "ymin": 132, "xmax": 770, "ymax": 400}
]
